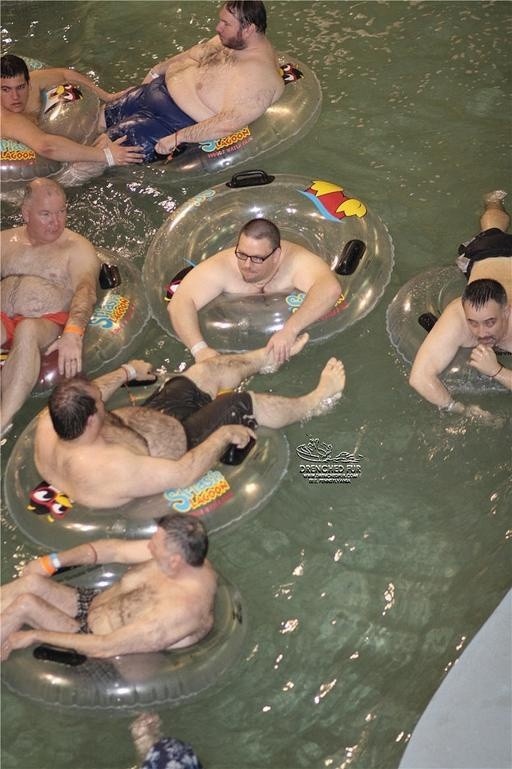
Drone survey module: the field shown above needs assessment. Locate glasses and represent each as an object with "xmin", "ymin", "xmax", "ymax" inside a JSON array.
[{"xmin": 235, "ymin": 242, "xmax": 281, "ymax": 264}]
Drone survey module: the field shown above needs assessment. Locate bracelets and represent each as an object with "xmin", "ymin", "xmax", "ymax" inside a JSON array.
[
  {"xmin": 103, "ymin": 148, "xmax": 115, "ymax": 168},
  {"xmin": 488, "ymin": 365, "xmax": 504, "ymax": 379},
  {"xmin": 42, "ymin": 552, "xmax": 61, "ymax": 574},
  {"xmin": 192, "ymin": 341, "xmax": 208, "ymax": 355},
  {"xmin": 121, "ymin": 363, "xmax": 136, "ymax": 382},
  {"xmin": 150, "ymin": 68, "xmax": 159, "ymax": 80},
  {"xmin": 62, "ymin": 326, "xmax": 84, "ymax": 337},
  {"xmin": 87, "ymin": 542, "xmax": 98, "ymax": 565},
  {"xmin": 441, "ymin": 398, "xmax": 458, "ymax": 415}
]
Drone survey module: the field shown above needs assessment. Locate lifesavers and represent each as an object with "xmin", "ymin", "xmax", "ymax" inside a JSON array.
[
  {"xmin": 0, "ymin": 48, "xmax": 99, "ymax": 194},
  {"xmin": 3, "ymin": 373, "xmax": 290, "ymax": 552},
  {"xmin": 144, "ymin": 170, "xmax": 395, "ymax": 354},
  {"xmin": 388, "ymin": 265, "xmax": 511, "ymax": 394},
  {"xmin": 1, "ymin": 226, "xmax": 148, "ymax": 398},
  {"xmin": 2, "ymin": 557, "xmax": 247, "ymax": 716},
  {"xmin": 103, "ymin": 51, "xmax": 323, "ymax": 186}
]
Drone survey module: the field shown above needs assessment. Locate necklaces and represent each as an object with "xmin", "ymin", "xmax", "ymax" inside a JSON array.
[{"xmin": 249, "ymin": 270, "xmax": 282, "ymax": 293}]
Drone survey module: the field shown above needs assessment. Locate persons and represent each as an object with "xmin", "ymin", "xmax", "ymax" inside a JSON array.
[
  {"xmin": 1, "ymin": 1, "xmax": 285, "ymax": 205},
  {"xmin": 34, "ymin": 332, "xmax": 347, "ymax": 507},
  {"xmin": 166, "ymin": 218, "xmax": 341, "ymax": 365},
  {"xmin": 0, "ymin": 177, "xmax": 100, "ymax": 433},
  {"xmin": 0, "ymin": 513, "xmax": 218, "ymax": 663},
  {"xmin": 410, "ymin": 191, "xmax": 512, "ymax": 418},
  {"xmin": 1, "ymin": 54, "xmax": 146, "ymax": 168}
]
